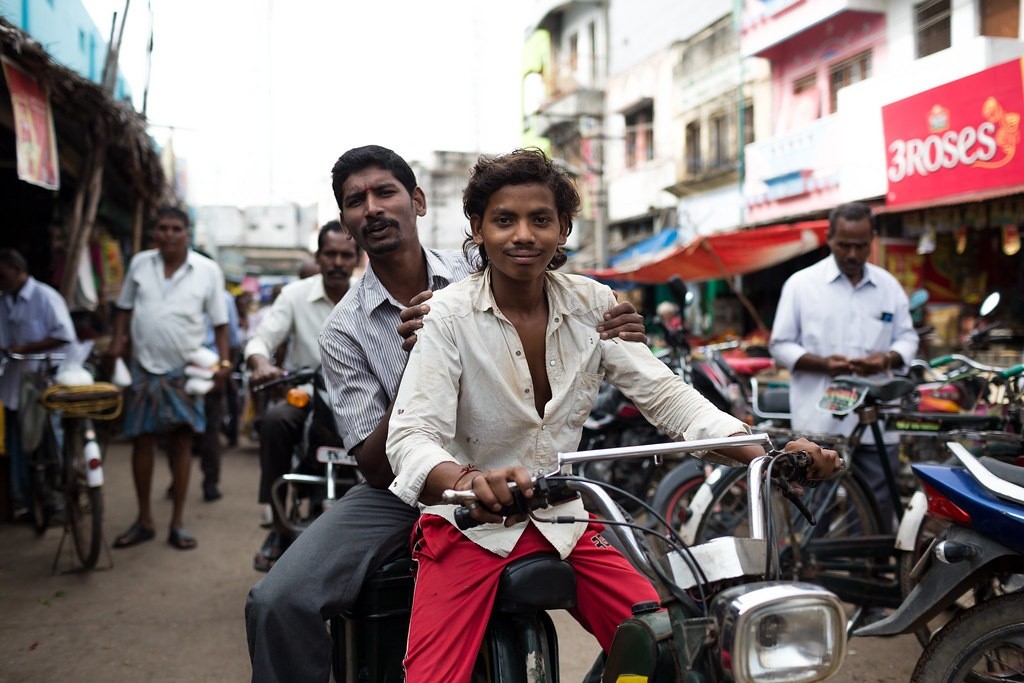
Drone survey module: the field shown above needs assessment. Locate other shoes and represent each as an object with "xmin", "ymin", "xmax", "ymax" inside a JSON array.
[
  {"xmin": 166, "ymin": 527, "xmax": 196, "ymax": 552},
  {"xmin": 114, "ymin": 521, "xmax": 156, "ymax": 552}
]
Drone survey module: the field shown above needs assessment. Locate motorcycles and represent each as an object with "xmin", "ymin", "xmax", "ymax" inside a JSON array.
[
  {"xmin": 327, "ymin": 435, "xmax": 855, "ymax": 683},
  {"xmin": 251, "ymin": 360, "xmax": 348, "ymax": 560},
  {"xmin": 852, "ymin": 438, "xmax": 1023, "ymax": 683}
]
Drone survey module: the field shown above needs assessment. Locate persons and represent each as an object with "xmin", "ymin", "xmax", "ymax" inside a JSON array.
[
  {"xmin": 3, "ymin": 252, "xmax": 76, "ymax": 519},
  {"xmin": 249, "ymin": 221, "xmax": 366, "ymax": 571},
  {"xmin": 382, "ymin": 147, "xmax": 840, "ymax": 681},
  {"xmin": 166, "ymin": 248, "xmax": 242, "ymax": 502},
  {"xmin": 242, "ymin": 143, "xmax": 650, "ymax": 680},
  {"xmin": 769, "ymin": 205, "xmax": 920, "ymax": 635},
  {"xmin": 107, "ymin": 205, "xmax": 230, "ymax": 553}
]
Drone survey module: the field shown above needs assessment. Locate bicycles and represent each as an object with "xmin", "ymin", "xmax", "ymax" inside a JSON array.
[
  {"xmin": 576, "ymin": 282, "xmax": 1024, "ymax": 648},
  {"xmin": 0, "ymin": 347, "xmax": 118, "ymax": 573}
]
